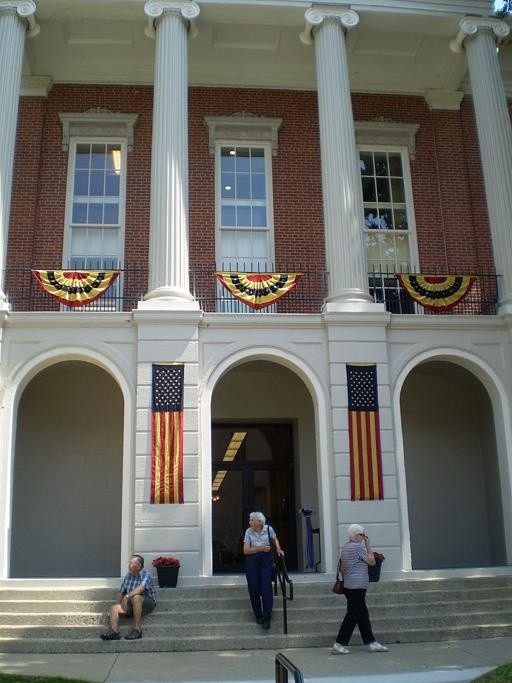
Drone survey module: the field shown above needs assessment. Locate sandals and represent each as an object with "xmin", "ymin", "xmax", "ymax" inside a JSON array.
[
  {"xmin": 100, "ymin": 629, "xmax": 122, "ymax": 641},
  {"xmin": 125, "ymin": 628, "xmax": 143, "ymax": 640}
]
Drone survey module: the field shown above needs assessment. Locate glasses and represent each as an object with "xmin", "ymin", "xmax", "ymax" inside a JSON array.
[{"xmin": 355, "ymin": 533, "xmax": 366, "ymax": 538}]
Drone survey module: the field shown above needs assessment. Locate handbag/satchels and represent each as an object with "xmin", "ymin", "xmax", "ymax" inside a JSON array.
[
  {"xmin": 332, "ymin": 557, "xmax": 345, "ymax": 594},
  {"xmin": 267, "ymin": 526, "xmax": 279, "ymax": 574}
]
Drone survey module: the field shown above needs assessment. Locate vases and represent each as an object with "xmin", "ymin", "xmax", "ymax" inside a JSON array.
[{"xmin": 156, "ymin": 566, "xmax": 180, "ymax": 588}]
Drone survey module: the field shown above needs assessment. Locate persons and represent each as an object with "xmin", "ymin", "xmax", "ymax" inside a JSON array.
[
  {"xmin": 243, "ymin": 511, "xmax": 286, "ymax": 630},
  {"xmin": 331, "ymin": 523, "xmax": 390, "ymax": 654},
  {"xmin": 98, "ymin": 554, "xmax": 158, "ymax": 640}
]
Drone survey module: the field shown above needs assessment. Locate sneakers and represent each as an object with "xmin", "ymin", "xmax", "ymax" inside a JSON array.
[
  {"xmin": 255, "ymin": 616, "xmax": 263, "ymax": 624},
  {"xmin": 261, "ymin": 620, "xmax": 271, "ymax": 630},
  {"xmin": 333, "ymin": 643, "xmax": 350, "ymax": 654},
  {"xmin": 369, "ymin": 643, "xmax": 388, "ymax": 652}
]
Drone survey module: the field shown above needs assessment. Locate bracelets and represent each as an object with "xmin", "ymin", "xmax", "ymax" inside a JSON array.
[{"xmin": 125, "ymin": 593, "xmax": 129, "ymax": 598}]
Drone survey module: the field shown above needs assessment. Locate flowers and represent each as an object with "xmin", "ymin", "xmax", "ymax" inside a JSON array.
[{"xmin": 151, "ymin": 556, "xmax": 179, "ymax": 567}]
